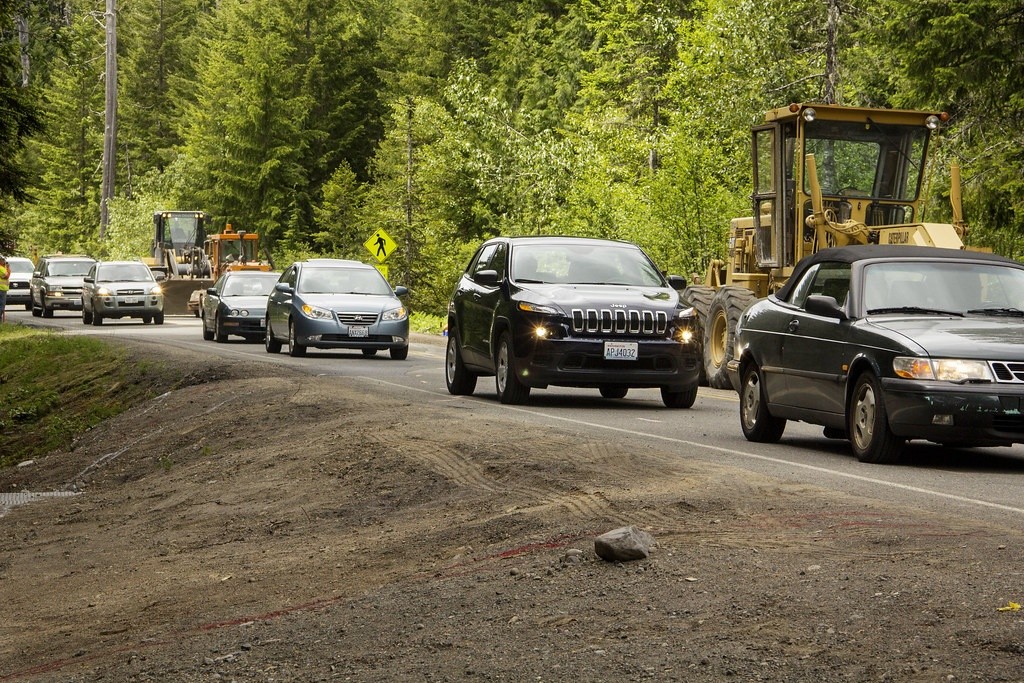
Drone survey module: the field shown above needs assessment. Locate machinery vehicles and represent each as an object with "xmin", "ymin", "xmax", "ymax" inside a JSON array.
[
  {"xmin": 682, "ymin": 102, "xmax": 994, "ymax": 391},
  {"xmin": 188, "ymin": 224, "xmax": 270, "ymax": 319},
  {"xmin": 142, "ymin": 209, "xmax": 206, "ymax": 316}
]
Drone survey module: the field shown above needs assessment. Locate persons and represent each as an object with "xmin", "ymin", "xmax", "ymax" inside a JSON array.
[{"xmin": 0, "ymin": 256, "xmax": 11, "ymax": 322}]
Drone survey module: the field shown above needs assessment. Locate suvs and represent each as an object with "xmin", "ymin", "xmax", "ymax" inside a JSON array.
[
  {"xmin": 5, "ymin": 257, "xmax": 35, "ymax": 311},
  {"xmin": 30, "ymin": 254, "xmax": 99, "ymax": 319},
  {"xmin": 82, "ymin": 260, "xmax": 164, "ymax": 326}
]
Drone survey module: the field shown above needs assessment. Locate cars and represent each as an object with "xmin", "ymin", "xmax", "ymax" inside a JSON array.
[
  {"xmin": 725, "ymin": 244, "xmax": 1024, "ymax": 462},
  {"xmin": 446, "ymin": 236, "xmax": 701, "ymax": 409},
  {"xmin": 200, "ymin": 270, "xmax": 282, "ymax": 343},
  {"xmin": 264, "ymin": 258, "xmax": 410, "ymax": 361}
]
